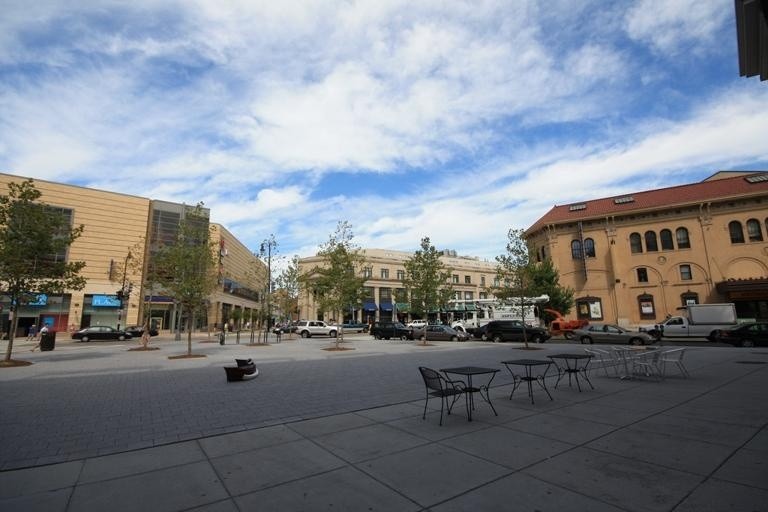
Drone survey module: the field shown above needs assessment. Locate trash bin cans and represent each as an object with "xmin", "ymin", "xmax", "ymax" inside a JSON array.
[{"xmin": 40, "ymin": 332, "xmax": 56, "ymax": 351}]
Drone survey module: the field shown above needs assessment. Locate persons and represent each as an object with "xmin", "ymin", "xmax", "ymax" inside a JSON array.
[
  {"xmin": 138, "ymin": 316, "xmax": 151, "ymax": 344},
  {"xmin": 655, "ymin": 325, "xmax": 664, "ymax": 338},
  {"xmin": 26, "ymin": 325, "xmax": 35, "ymax": 341},
  {"xmin": 367, "ymin": 314, "xmax": 372, "ymax": 333},
  {"xmin": 213, "ymin": 318, "xmax": 288, "ymax": 345},
  {"xmin": 653, "ymin": 325, "xmax": 664, "ymax": 346},
  {"xmin": 476, "ymin": 301, "xmax": 481, "ymax": 313},
  {"xmin": 29, "ymin": 322, "xmax": 49, "ymax": 352}
]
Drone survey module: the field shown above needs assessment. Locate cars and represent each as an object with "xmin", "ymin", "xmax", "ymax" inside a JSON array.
[
  {"xmin": 412, "ymin": 325, "xmax": 470, "ymax": 342},
  {"xmin": 71, "ymin": 325, "xmax": 132, "ymax": 342},
  {"xmin": 272, "ymin": 321, "xmax": 297, "ymax": 334},
  {"xmin": 573, "ymin": 322, "xmax": 657, "ymax": 347},
  {"xmin": 121, "ymin": 326, "xmax": 158, "ymax": 338},
  {"xmin": 705, "ymin": 322, "xmax": 768, "ymax": 346},
  {"xmin": 470, "ymin": 323, "xmax": 488, "ymax": 341}
]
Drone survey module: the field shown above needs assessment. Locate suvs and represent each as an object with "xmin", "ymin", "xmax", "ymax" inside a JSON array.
[
  {"xmin": 544, "ymin": 309, "xmax": 591, "ymax": 340},
  {"xmin": 370, "ymin": 321, "xmax": 414, "ymax": 342},
  {"xmin": 296, "ymin": 320, "xmax": 341, "ymax": 338},
  {"xmin": 485, "ymin": 320, "xmax": 552, "ymax": 344},
  {"xmin": 406, "ymin": 319, "xmax": 429, "ymax": 329}
]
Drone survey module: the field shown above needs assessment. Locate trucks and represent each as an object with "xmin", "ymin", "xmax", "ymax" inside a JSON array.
[{"xmin": 450, "ymin": 305, "xmax": 536, "ymax": 334}]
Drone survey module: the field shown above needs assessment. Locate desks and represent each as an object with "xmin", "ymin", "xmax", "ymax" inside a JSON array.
[{"xmin": 436, "ymin": 354, "xmax": 596, "ymax": 422}]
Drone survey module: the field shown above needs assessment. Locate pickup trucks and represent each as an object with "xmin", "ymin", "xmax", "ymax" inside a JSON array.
[
  {"xmin": 638, "ymin": 303, "xmax": 741, "ymax": 342},
  {"xmin": 333, "ymin": 320, "xmax": 369, "ymax": 333}
]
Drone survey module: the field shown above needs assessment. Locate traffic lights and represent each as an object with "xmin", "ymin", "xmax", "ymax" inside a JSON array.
[
  {"xmin": 116, "ymin": 290, "xmax": 123, "ymax": 300},
  {"xmin": 126, "ymin": 281, "xmax": 133, "ymax": 292}
]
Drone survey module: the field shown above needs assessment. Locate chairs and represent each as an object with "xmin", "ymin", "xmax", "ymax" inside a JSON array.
[
  {"xmin": 417, "ymin": 365, "xmax": 473, "ymax": 425},
  {"xmin": 583, "ymin": 344, "xmax": 691, "ymax": 384}
]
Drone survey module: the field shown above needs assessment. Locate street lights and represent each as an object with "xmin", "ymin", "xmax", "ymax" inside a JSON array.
[
  {"xmin": 258, "ymin": 242, "xmax": 271, "ymax": 332},
  {"xmin": 117, "ymin": 251, "xmax": 133, "ymax": 331}
]
